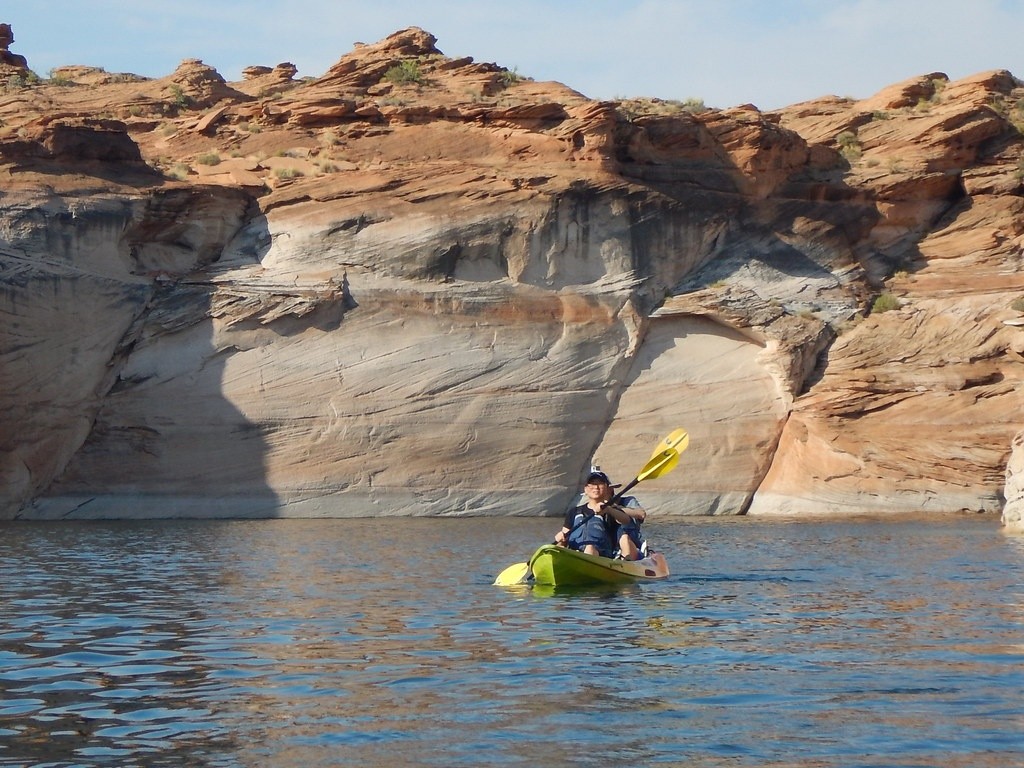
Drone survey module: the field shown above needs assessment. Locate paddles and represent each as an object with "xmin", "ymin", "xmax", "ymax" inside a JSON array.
[
  {"xmin": 552, "ymin": 448, "xmax": 681, "ymax": 543},
  {"xmin": 492, "ymin": 426, "xmax": 691, "ymax": 585}
]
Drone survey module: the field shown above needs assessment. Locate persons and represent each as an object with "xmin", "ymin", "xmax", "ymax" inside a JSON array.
[
  {"xmin": 604, "ymin": 481, "xmax": 647, "ymax": 561},
  {"xmin": 554, "ymin": 466, "xmax": 630, "ymax": 557}
]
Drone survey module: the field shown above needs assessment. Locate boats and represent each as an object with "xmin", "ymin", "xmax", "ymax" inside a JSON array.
[{"xmin": 528, "ymin": 543, "xmax": 671, "ymax": 588}]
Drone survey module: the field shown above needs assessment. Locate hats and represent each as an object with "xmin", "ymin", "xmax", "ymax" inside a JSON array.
[
  {"xmin": 607, "ymin": 479, "xmax": 622, "ymax": 488},
  {"xmin": 585, "ymin": 472, "xmax": 609, "ymax": 487}
]
